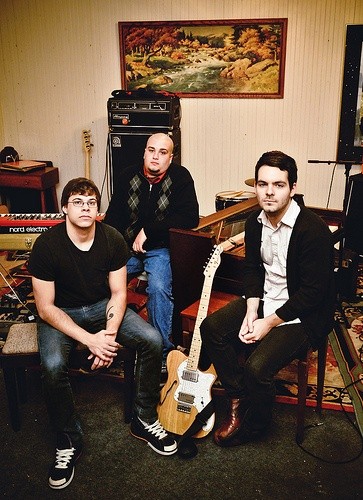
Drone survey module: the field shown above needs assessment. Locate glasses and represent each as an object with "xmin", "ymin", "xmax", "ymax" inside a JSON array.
[{"xmin": 67, "ymin": 199, "xmax": 98, "ymax": 208}]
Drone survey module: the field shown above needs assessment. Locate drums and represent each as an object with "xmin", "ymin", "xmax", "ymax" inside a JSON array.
[{"xmin": 215, "ymin": 191, "xmax": 256, "ymax": 221}]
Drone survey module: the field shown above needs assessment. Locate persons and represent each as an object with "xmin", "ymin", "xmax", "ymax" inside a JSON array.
[
  {"xmin": 27, "ymin": 177, "xmax": 178, "ymax": 489},
  {"xmin": 199, "ymin": 151, "xmax": 335, "ymax": 447},
  {"xmin": 79, "ymin": 133, "xmax": 199, "ymax": 376}
]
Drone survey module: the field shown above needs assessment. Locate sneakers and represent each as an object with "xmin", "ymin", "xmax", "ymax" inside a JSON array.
[
  {"xmin": 48, "ymin": 447, "xmax": 83, "ymax": 490},
  {"xmin": 129, "ymin": 415, "xmax": 178, "ymax": 456}
]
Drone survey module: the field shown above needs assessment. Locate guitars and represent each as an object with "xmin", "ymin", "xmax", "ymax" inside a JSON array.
[
  {"xmin": 82, "ymin": 128, "xmax": 94, "ymax": 178},
  {"xmin": 155, "ymin": 244, "xmax": 224, "ymax": 438}
]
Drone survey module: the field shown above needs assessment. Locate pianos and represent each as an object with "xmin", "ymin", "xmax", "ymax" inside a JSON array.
[{"xmin": 0, "ymin": 212, "xmax": 107, "ymax": 234}]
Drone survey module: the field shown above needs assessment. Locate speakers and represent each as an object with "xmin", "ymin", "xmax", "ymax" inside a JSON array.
[{"xmin": 109, "ymin": 129, "xmax": 181, "ymax": 198}]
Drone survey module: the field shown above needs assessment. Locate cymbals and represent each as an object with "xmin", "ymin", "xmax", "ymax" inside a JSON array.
[{"xmin": 245, "ymin": 179, "xmax": 254, "ymax": 187}]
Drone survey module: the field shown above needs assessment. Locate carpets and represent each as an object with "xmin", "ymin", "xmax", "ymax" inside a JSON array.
[{"xmin": 0, "ymin": 248, "xmax": 363, "ymax": 438}]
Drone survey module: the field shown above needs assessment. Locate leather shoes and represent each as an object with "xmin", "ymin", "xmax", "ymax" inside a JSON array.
[{"xmin": 218, "ymin": 394, "xmax": 262, "ymax": 442}]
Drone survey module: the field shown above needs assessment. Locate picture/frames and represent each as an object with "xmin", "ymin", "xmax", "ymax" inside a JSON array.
[{"xmin": 118, "ymin": 18, "xmax": 288, "ymax": 99}]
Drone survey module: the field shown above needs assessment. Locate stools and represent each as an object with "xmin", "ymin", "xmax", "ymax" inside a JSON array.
[
  {"xmin": 180, "ymin": 291, "xmax": 241, "ymax": 396},
  {"xmin": 273, "ymin": 336, "xmax": 329, "ymax": 445},
  {"xmin": 0, "ymin": 323, "xmax": 136, "ymax": 432},
  {"xmin": 0, "ymin": 167, "xmax": 59, "ymax": 214}
]
